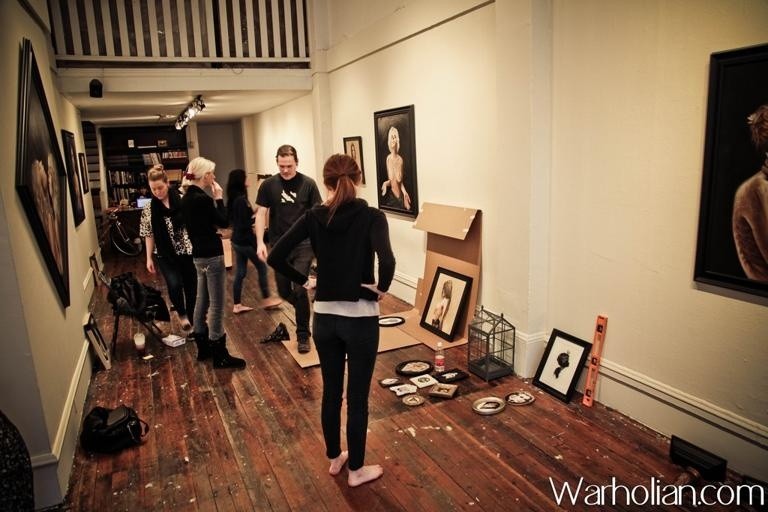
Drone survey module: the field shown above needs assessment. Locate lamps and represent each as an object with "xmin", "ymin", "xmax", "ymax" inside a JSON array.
[{"xmin": 172, "ymin": 93, "xmax": 206, "ymax": 133}]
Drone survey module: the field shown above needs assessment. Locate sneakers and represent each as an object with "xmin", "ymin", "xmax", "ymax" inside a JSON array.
[
  {"xmin": 298, "ymin": 337, "xmax": 310, "ymax": 352},
  {"xmin": 187, "ymin": 326, "xmax": 196, "ymax": 341},
  {"xmin": 177, "ymin": 314, "xmax": 191, "ymax": 329}
]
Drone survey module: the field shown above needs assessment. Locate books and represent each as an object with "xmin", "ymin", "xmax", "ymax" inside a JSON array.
[{"xmin": 109, "ymin": 151, "xmax": 182, "ymax": 202}]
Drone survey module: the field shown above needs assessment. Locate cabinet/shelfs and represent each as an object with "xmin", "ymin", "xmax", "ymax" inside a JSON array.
[{"xmin": 100, "ymin": 123, "xmax": 188, "ymax": 207}]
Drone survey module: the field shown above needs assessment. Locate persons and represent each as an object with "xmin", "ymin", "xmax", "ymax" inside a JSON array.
[
  {"xmin": 731, "ymin": 106, "xmax": 768, "ymax": 282},
  {"xmin": 140, "ymin": 163, "xmax": 199, "ymax": 342},
  {"xmin": 508, "ymin": 392, "xmax": 533, "ymax": 404},
  {"xmin": 30, "ymin": 152, "xmax": 66, "ymax": 276},
  {"xmin": 431, "ymin": 280, "xmax": 452, "ymax": 331},
  {"xmin": 264, "ymin": 153, "xmax": 399, "ymax": 486},
  {"xmin": 225, "ymin": 166, "xmax": 284, "ymax": 314},
  {"xmin": 253, "ymin": 143, "xmax": 324, "ymax": 352},
  {"xmin": 69, "ymin": 146, "xmax": 84, "ymax": 221},
  {"xmin": 350, "ymin": 144, "xmax": 357, "ymax": 160},
  {"xmin": 381, "ymin": 126, "xmax": 411, "ymax": 212},
  {"xmin": 178, "ymin": 156, "xmax": 247, "ymax": 369}
]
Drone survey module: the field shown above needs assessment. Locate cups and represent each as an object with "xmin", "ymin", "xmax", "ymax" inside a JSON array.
[{"xmin": 132, "ymin": 334, "xmax": 145, "ymax": 350}]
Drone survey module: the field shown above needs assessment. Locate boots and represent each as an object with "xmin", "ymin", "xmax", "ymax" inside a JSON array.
[
  {"xmin": 193, "ymin": 327, "xmax": 210, "ymax": 361},
  {"xmin": 209, "ymin": 332, "xmax": 246, "ymax": 368}
]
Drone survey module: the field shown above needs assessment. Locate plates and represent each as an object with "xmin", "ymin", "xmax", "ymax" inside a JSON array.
[
  {"xmin": 430, "ymin": 367, "xmax": 469, "ymax": 384},
  {"xmin": 504, "ymin": 391, "xmax": 535, "ymax": 406},
  {"xmin": 427, "ymin": 383, "xmax": 458, "ymax": 399},
  {"xmin": 470, "ymin": 397, "xmax": 505, "ymax": 416},
  {"xmin": 402, "ymin": 395, "xmax": 425, "ymax": 407},
  {"xmin": 408, "ymin": 373, "xmax": 438, "ymax": 389},
  {"xmin": 395, "ymin": 359, "xmax": 433, "ymax": 377}
]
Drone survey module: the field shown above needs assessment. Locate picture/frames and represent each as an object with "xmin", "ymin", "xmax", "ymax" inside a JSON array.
[
  {"xmin": 530, "ymin": 327, "xmax": 595, "ymax": 401},
  {"xmin": 692, "ymin": 41, "xmax": 768, "ymax": 306},
  {"xmin": 60, "ymin": 128, "xmax": 87, "ymax": 228},
  {"xmin": 78, "ymin": 153, "xmax": 89, "ymax": 194},
  {"xmin": 81, "ymin": 313, "xmax": 114, "ymax": 371},
  {"xmin": 372, "ymin": 102, "xmax": 421, "ymax": 218},
  {"xmin": 417, "ymin": 267, "xmax": 473, "ymax": 341},
  {"xmin": 342, "ymin": 135, "xmax": 366, "ymax": 185},
  {"xmin": 378, "ymin": 314, "xmax": 406, "ymax": 328},
  {"xmin": 377, "ymin": 359, "xmax": 535, "ymax": 416},
  {"xmin": 14, "ymin": 37, "xmax": 71, "ymax": 309}
]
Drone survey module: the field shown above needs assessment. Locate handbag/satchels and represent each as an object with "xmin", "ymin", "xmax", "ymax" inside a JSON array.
[
  {"xmin": 80, "ymin": 404, "xmax": 149, "ymax": 452},
  {"xmin": 107, "ymin": 271, "xmax": 146, "ymax": 311}
]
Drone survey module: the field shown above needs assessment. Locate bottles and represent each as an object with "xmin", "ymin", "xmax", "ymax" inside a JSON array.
[{"xmin": 433, "ymin": 342, "xmax": 445, "ymax": 373}]
